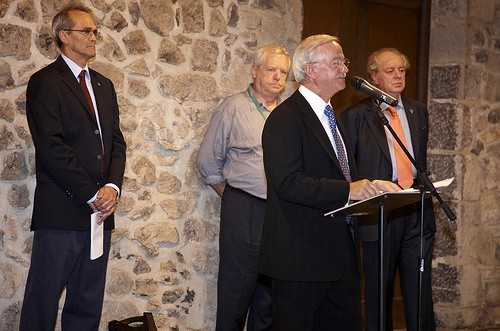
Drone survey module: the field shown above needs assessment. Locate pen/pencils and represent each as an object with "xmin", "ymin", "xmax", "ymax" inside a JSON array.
[{"xmin": 379, "ymin": 190, "xmax": 385, "ymax": 194}]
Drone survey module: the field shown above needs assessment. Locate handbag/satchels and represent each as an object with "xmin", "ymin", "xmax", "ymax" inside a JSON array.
[{"xmin": 108, "ymin": 312, "xmax": 157, "ymax": 331}]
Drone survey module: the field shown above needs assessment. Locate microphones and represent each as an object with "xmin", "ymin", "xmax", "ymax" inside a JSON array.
[{"xmin": 350, "ymin": 76, "xmax": 399, "ymax": 108}]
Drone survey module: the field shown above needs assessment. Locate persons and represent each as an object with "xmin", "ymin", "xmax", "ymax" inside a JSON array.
[
  {"xmin": 195, "ymin": 42, "xmax": 292, "ymax": 330},
  {"xmin": 17, "ymin": 4, "xmax": 128, "ymax": 331},
  {"xmin": 334, "ymin": 47, "xmax": 437, "ymax": 331},
  {"xmin": 260, "ymin": 33, "xmax": 362, "ymax": 331}
]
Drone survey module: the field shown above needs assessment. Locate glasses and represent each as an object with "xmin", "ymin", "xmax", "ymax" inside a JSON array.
[
  {"xmin": 310, "ymin": 57, "xmax": 350, "ymax": 70},
  {"xmin": 64, "ymin": 29, "xmax": 100, "ymax": 40}
]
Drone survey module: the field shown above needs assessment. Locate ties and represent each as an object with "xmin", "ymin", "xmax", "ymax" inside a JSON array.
[
  {"xmin": 324, "ymin": 104, "xmax": 352, "ymax": 225},
  {"xmin": 387, "ymin": 105, "xmax": 414, "ymax": 189},
  {"xmin": 78, "ymin": 69, "xmax": 104, "ymax": 178}
]
